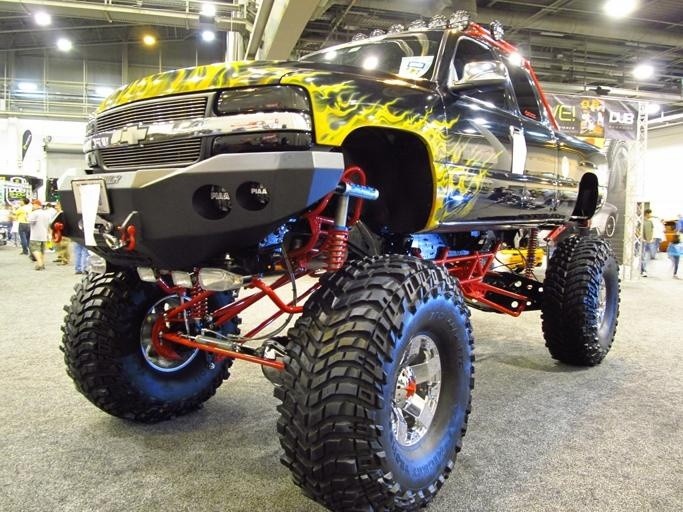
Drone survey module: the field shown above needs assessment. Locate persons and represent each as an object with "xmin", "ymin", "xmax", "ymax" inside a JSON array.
[
  {"xmin": 73, "ymin": 241, "xmax": 91, "ymax": 274},
  {"xmin": 667, "ymin": 230, "xmax": 683, "ymax": 277},
  {"xmin": 637, "ymin": 208, "xmax": 655, "ymax": 276},
  {"xmin": 9, "ymin": 196, "xmax": 69, "ymax": 270}
]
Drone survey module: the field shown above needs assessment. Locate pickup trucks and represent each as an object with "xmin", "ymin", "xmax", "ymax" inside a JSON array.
[{"xmin": 49, "ymin": 8, "xmax": 621, "ymax": 512}]
